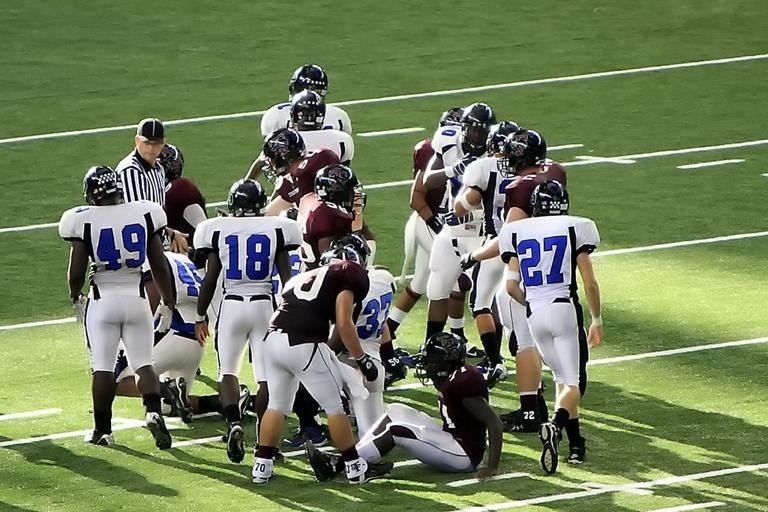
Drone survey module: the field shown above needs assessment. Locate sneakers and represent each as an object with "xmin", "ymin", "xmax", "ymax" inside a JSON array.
[
  {"xmin": 345, "ymin": 457, "xmax": 394, "ymax": 486},
  {"xmin": 83, "ymin": 428, "xmax": 115, "ymax": 447},
  {"xmin": 501, "ymin": 388, "xmax": 544, "ymax": 435},
  {"xmin": 222, "ymin": 422, "xmax": 247, "ymax": 465},
  {"xmin": 485, "ymin": 362, "xmax": 509, "ymax": 389},
  {"xmin": 566, "ymin": 437, "xmax": 589, "ymax": 467},
  {"xmin": 466, "ymin": 345, "xmax": 486, "ymax": 357},
  {"xmin": 238, "ymin": 384, "xmax": 251, "ymax": 422},
  {"xmin": 163, "ymin": 376, "xmax": 198, "ymax": 424},
  {"xmin": 283, "ymin": 423, "xmax": 330, "ymax": 448},
  {"xmin": 304, "ymin": 438, "xmax": 346, "ymax": 483},
  {"xmin": 475, "ymin": 356, "xmax": 490, "ymax": 372},
  {"xmin": 538, "ymin": 421, "xmax": 564, "ymax": 474},
  {"xmin": 144, "ymin": 410, "xmax": 172, "ymax": 452},
  {"xmin": 498, "ymin": 395, "xmax": 549, "ymax": 424},
  {"xmin": 251, "ymin": 446, "xmax": 276, "ymax": 485}
]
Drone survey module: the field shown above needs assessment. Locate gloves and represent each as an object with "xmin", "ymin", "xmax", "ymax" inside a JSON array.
[
  {"xmin": 71, "ymin": 293, "xmax": 90, "ymax": 326},
  {"xmin": 354, "ymin": 353, "xmax": 379, "ymax": 384},
  {"xmin": 425, "ymin": 214, "xmax": 444, "ymax": 234},
  {"xmin": 153, "ymin": 303, "xmax": 173, "ymax": 334},
  {"xmin": 459, "ymin": 252, "xmax": 478, "ymax": 272}
]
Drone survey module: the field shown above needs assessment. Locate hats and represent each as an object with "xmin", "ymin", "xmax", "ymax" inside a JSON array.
[{"xmin": 137, "ymin": 118, "xmax": 166, "ymax": 142}]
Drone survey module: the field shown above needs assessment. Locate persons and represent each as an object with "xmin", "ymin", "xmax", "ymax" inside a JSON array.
[
  {"xmin": 116, "ymin": 118, "xmax": 190, "ymax": 255},
  {"xmin": 386, "ymin": 107, "xmax": 486, "ymax": 357},
  {"xmin": 306, "ymin": 333, "xmax": 503, "ymax": 481},
  {"xmin": 424, "ymin": 103, "xmax": 504, "ymax": 372},
  {"xmin": 115, "ymin": 226, "xmax": 249, "ymax": 423},
  {"xmin": 59, "ymin": 165, "xmax": 173, "ymax": 449},
  {"xmin": 461, "ymin": 129, "xmax": 566, "ymax": 432},
  {"xmin": 283, "ymin": 235, "xmax": 394, "ymax": 447},
  {"xmin": 195, "ymin": 179, "xmax": 290, "ymax": 467},
  {"xmin": 456, "ymin": 121, "xmax": 519, "ymax": 388},
  {"xmin": 261, "ymin": 64, "xmax": 352, "ymax": 136},
  {"xmin": 156, "ymin": 143, "xmax": 208, "ymax": 263},
  {"xmin": 263, "ymin": 128, "xmax": 375, "ymax": 266},
  {"xmin": 244, "ymin": 90, "xmax": 355, "ymax": 219},
  {"xmin": 499, "ymin": 181, "xmax": 603, "ymax": 473},
  {"xmin": 296, "ymin": 164, "xmax": 367, "ymax": 271},
  {"xmin": 252, "ymin": 247, "xmax": 395, "ymax": 485}
]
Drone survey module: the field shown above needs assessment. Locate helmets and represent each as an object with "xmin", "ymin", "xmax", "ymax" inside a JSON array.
[
  {"xmin": 532, "ymin": 180, "xmax": 570, "ymax": 217},
  {"xmin": 438, "ymin": 107, "xmax": 466, "ymax": 130},
  {"xmin": 158, "ymin": 226, "xmax": 172, "ymax": 251},
  {"xmin": 288, "ymin": 63, "xmax": 330, "ymax": 100},
  {"xmin": 414, "ymin": 334, "xmax": 467, "ymax": 386},
  {"xmin": 262, "ymin": 128, "xmax": 307, "ymax": 184},
  {"xmin": 290, "ymin": 90, "xmax": 326, "ymax": 131},
  {"xmin": 461, "ymin": 102, "xmax": 496, "ymax": 156},
  {"xmin": 496, "ymin": 130, "xmax": 546, "ymax": 178},
  {"xmin": 486, "ymin": 119, "xmax": 520, "ymax": 155},
  {"xmin": 226, "ymin": 178, "xmax": 269, "ymax": 217},
  {"xmin": 315, "ymin": 165, "xmax": 368, "ymax": 214},
  {"xmin": 158, "ymin": 143, "xmax": 185, "ymax": 182},
  {"xmin": 318, "ymin": 244, "xmax": 365, "ymax": 268},
  {"xmin": 329, "ymin": 234, "xmax": 372, "ymax": 269},
  {"xmin": 82, "ymin": 165, "xmax": 125, "ymax": 206}
]
